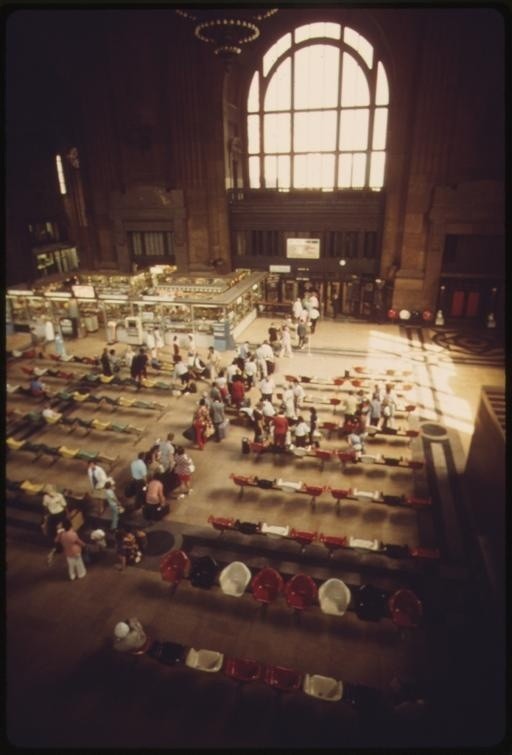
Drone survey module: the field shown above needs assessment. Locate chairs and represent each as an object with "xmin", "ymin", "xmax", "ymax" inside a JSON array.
[
  {"xmin": 5, "ymin": 357, "xmax": 442, "ymax": 731},
  {"xmin": 384, "ymin": 305, "xmax": 436, "ymax": 325}
]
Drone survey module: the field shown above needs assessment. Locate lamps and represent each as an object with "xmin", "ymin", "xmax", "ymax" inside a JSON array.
[{"xmin": 175, "ymin": 0, "xmax": 277, "ymax": 68}]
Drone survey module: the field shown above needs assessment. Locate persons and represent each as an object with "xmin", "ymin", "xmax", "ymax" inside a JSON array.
[
  {"xmin": 113, "ymin": 616, "xmax": 147, "ymax": 653},
  {"xmin": 268, "ymin": 294, "xmax": 321, "ymax": 358},
  {"xmin": 128, "ymin": 432, "xmax": 195, "ymax": 519},
  {"xmin": 43, "ymin": 318, "xmax": 63, "ymax": 356},
  {"xmin": 253, "ymin": 373, "xmax": 316, "ymax": 449},
  {"xmin": 101, "ymin": 327, "xmax": 196, "ymax": 385},
  {"xmin": 343, "ymin": 384, "xmax": 395, "ymax": 460},
  {"xmin": 41, "ymin": 458, "xmax": 151, "ymax": 579},
  {"xmin": 31, "ymin": 375, "xmax": 46, "ymax": 398},
  {"xmin": 193, "ymin": 339, "xmax": 274, "ymax": 449}
]
